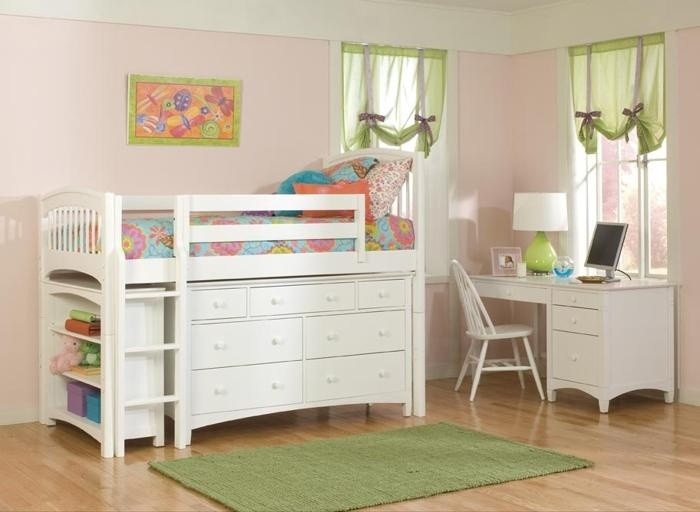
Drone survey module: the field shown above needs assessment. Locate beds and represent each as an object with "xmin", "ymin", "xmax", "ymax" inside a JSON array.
[{"xmin": 40, "ymin": 148, "xmax": 426, "ymax": 460}]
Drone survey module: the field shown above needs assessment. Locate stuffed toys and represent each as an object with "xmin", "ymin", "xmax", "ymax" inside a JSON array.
[
  {"xmin": 80, "ymin": 341, "xmax": 102, "ymax": 367},
  {"xmin": 47, "ymin": 336, "xmax": 83, "ymax": 375}
]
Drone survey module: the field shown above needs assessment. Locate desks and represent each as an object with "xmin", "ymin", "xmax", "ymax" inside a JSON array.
[{"xmin": 465, "ymin": 274, "xmax": 676, "ymax": 414}]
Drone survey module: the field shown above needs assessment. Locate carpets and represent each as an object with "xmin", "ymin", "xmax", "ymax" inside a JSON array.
[{"xmin": 149, "ymin": 422, "xmax": 596, "ymax": 512}]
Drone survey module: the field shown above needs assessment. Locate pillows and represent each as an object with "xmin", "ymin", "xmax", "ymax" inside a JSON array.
[
  {"xmin": 241, "ymin": 158, "xmax": 331, "ymax": 217},
  {"xmin": 318, "ymin": 156, "xmax": 380, "ymax": 184},
  {"xmin": 364, "ymin": 159, "xmax": 414, "ymax": 221},
  {"xmin": 290, "ymin": 181, "xmax": 377, "ymax": 225}
]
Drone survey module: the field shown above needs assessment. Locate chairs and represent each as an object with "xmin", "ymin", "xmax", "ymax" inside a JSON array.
[{"xmin": 449, "ymin": 258, "xmax": 548, "ymax": 402}]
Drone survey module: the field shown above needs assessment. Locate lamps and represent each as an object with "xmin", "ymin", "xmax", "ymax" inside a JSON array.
[{"xmin": 512, "ymin": 192, "xmax": 568, "ymax": 277}]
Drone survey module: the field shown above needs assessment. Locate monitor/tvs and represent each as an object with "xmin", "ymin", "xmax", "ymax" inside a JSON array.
[{"xmin": 584, "ymin": 221, "xmax": 629, "ymax": 282}]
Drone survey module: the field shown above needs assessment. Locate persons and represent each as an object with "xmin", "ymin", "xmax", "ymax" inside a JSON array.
[{"xmin": 500, "ymin": 256, "xmax": 514, "ymax": 269}]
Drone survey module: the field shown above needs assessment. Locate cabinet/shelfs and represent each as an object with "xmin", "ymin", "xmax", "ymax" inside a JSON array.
[
  {"xmin": 187, "ymin": 271, "xmax": 413, "ymax": 444},
  {"xmin": 41, "ymin": 279, "xmax": 178, "ymax": 459}
]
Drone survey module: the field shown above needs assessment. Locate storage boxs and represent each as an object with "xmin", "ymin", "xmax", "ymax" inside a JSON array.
[
  {"xmin": 86, "ymin": 392, "xmax": 101, "ymax": 423},
  {"xmin": 67, "ymin": 382, "xmax": 98, "ymax": 418}
]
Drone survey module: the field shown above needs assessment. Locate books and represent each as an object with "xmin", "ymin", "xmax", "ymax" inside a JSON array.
[
  {"xmin": 65, "ymin": 319, "xmax": 100, "ymax": 336},
  {"xmin": 73, "ymin": 367, "xmax": 100, "ymax": 376},
  {"xmin": 75, "ymin": 365, "xmax": 100, "ymax": 371},
  {"xmin": 70, "ymin": 309, "xmax": 100, "ymax": 323}
]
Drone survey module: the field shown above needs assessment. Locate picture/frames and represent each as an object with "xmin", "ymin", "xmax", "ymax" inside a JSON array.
[
  {"xmin": 127, "ymin": 73, "xmax": 241, "ymax": 148},
  {"xmin": 489, "ymin": 247, "xmax": 524, "ymax": 277}
]
